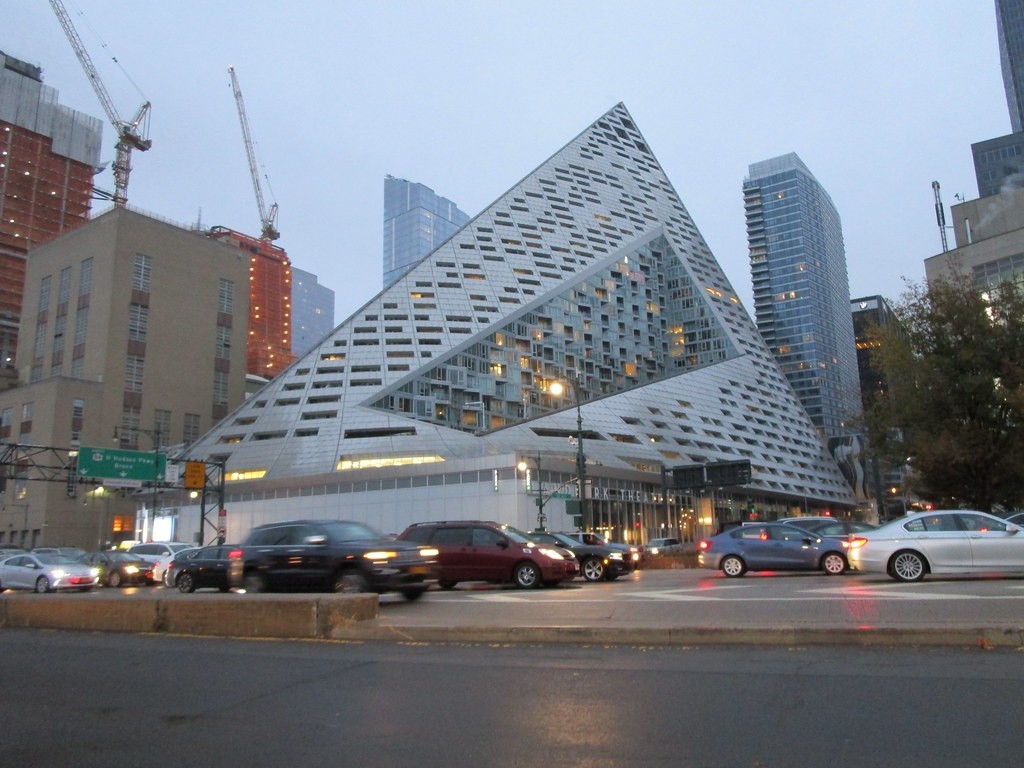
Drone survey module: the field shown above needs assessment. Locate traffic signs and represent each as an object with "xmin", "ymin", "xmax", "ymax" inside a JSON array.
[{"xmin": 77, "ymin": 447, "xmax": 166, "ymax": 482}]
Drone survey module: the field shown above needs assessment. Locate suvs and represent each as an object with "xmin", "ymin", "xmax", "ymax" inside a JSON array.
[
  {"xmin": 564, "ymin": 532, "xmax": 647, "ymax": 563},
  {"xmin": 527, "ymin": 532, "xmax": 627, "ymax": 581},
  {"xmin": 227, "ymin": 519, "xmax": 440, "ymax": 602},
  {"xmin": 392, "ymin": 520, "xmax": 579, "ymax": 590},
  {"xmin": 127, "ymin": 542, "xmax": 193, "ymax": 564},
  {"xmin": 777, "ymin": 516, "xmax": 840, "ymax": 533},
  {"xmin": 646, "ymin": 537, "xmax": 682, "ymax": 559}
]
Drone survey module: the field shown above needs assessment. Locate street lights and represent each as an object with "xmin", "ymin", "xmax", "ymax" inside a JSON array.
[
  {"xmin": 518, "ymin": 451, "xmax": 544, "ymax": 529},
  {"xmin": 549, "ymin": 370, "xmax": 589, "ymax": 529}
]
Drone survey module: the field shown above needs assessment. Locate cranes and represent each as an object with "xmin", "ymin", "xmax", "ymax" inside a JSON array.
[
  {"xmin": 49, "ymin": 0, "xmax": 154, "ymax": 213},
  {"xmin": 227, "ymin": 65, "xmax": 282, "ymax": 244}
]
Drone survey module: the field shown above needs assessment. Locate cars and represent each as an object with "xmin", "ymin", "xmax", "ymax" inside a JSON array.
[
  {"xmin": 0, "ymin": 552, "xmax": 98, "ymax": 593},
  {"xmin": 977, "ymin": 509, "xmax": 1024, "ymax": 537},
  {"xmin": 696, "ymin": 524, "xmax": 850, "ymax": 578},
  {"xmin": 0, "ymin": 542, "xmax": 87, "ymax": 563},
  {"xmin": 76, "ymin": 550, "xmax": 159, "ymax": 589},
  {"xmin": 165, "ymin": 545, "xmax": 242, "ymax": 595},
  {"xmin": 846, "ymin": 509, "xmax": 1023, "ymax": 582},
  {"xmin": 808, "ymin": 521, "xmax": 881, "ymax": 544},
  {"xmin": 154, "ymin": 548, "xmax": 203, "ymax": 586}
]
